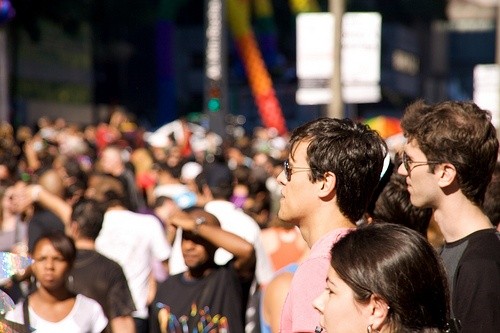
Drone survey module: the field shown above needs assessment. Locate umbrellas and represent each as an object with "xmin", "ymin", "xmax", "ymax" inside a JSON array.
[{"xmin": 147, "ymin": 119, "xmax": 203, "ymax": 144}]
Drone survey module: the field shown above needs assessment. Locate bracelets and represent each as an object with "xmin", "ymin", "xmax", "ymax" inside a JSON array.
[
  {"xmin": 194, "ymin": 218, "xmax": 206, "ymax": 233},
  {"xmin": 32, "ymin": 185, "xmax": 41, "ymax": 198}
]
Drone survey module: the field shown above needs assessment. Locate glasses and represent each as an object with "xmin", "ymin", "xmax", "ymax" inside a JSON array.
[
  {"xmin": 284, "ymin": 161, "xmax": 316, "ymax": 180},
  {"xmin": 403, "ymin": 152, "xmax": 442, "ymax": 172}
]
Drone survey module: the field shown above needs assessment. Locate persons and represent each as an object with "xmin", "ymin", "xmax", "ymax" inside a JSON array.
[
  {"xmin": 277, "ymin": 117, "xmax": 394, "ymax": 333},
  {"xmin": 0, "ymin": 110, "xmax": 500, "ymax": 333},
  {"xmin": 313, "ymin": 224, "xmax": 457, "ymax": 333},
  {"xmin": 7, "ymin": 233, "xmax": 108, "ymax": 333},
  {"xmin": 151, "ymin": 207, "xmax": 257, "ymax": 333},
  {"xmin": 170, "ymin": 166, "xmax": 273, "ymax": 284},
  {"xmin": 65, "ymin": 200, "xmax": 136, "ymax": 333},
  {"xmin": 398, "ymin": 99, "xmax": 500, "ymax": 333},
  {"xmin": 94, "ymin": 176, "xmax": 169, "ymax": 333}
]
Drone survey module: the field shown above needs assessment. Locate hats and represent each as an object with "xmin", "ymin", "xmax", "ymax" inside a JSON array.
[{"xmin": 204, "ymin": 165, "xmax": 234, "ymax": 187}]
